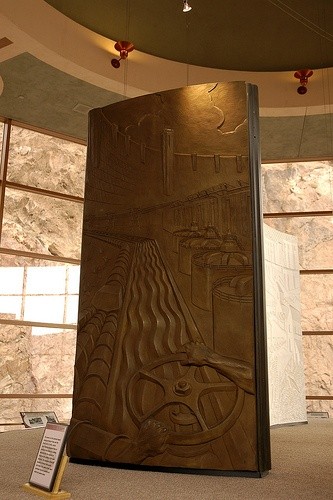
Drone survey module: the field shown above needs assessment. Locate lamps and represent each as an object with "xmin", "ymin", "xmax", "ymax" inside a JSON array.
[
  {"xmin": 183, "ymin": 0, "xmax": 192, "ymax": 13},
  {"xmin": 292, "ymin": 69, "xmax": 313, "ymax": 95},
  {"xmin": 111, "ymin": 40, "xmax": 136, "ymax": 68}
]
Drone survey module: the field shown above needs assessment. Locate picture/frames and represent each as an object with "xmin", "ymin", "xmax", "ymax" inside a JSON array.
[{"xmin": 28, "ymin": 421, "xmax": 71, "ymax": 493}]
